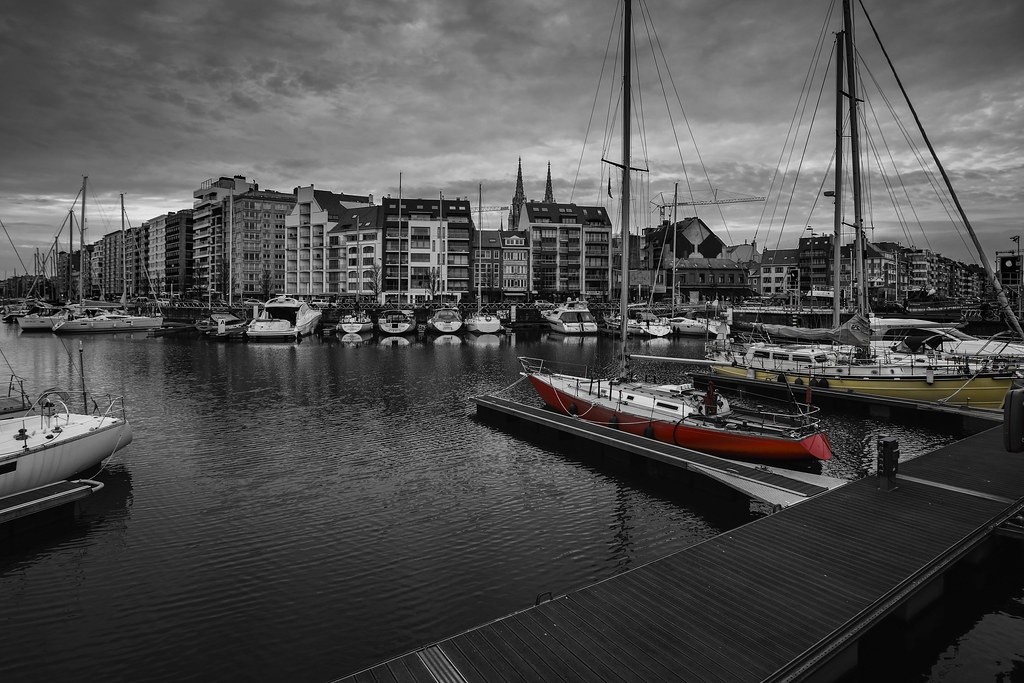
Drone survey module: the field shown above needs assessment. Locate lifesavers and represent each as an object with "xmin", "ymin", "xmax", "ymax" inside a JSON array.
[{"xmin": 485, "ymin": 315, "xmax": 492, "ymax": 322}]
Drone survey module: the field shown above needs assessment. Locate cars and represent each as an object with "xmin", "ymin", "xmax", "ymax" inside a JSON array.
[
  {"xmin": 533, "ymin": 299, "xmax": 557, "ymax": 310},
  {"xmin": 885, "ymin": 302, "xmax": 904, "ymax": 313}
]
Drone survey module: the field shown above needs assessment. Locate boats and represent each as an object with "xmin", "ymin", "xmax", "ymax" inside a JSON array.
[
  {"xmin": 1, "ymin": 222, "xmax": 132, "ymax": 544},
  {"xmin": 246, "ymin": 295, "xmax": 323, "ymax": 341}
]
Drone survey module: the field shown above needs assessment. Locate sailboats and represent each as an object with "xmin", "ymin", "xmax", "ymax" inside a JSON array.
[
  {"xmin": 515, "ymin": 1, "xmax": 833, "ymax": 465},
  {"xmin": 462, "ymin": 180, "xmax": 505, "ymax": 335},
  {"xmin": 538, "ymin": 0, "xmax": 1024, "ymax": 431},
  {"xmin": 425, "ymin": 190, "xmax": 464, "ymax": 335},
  {"xmin": 1, "ymin": 172, "xmax": 247, "ymax": 341},
  {"xmin": 334, "ymin": 214, "xmax": 374, "ymax": 335},
  {"xmin": 377, "ymin": 170, "xmax": 416, "ymax": 335}
]
